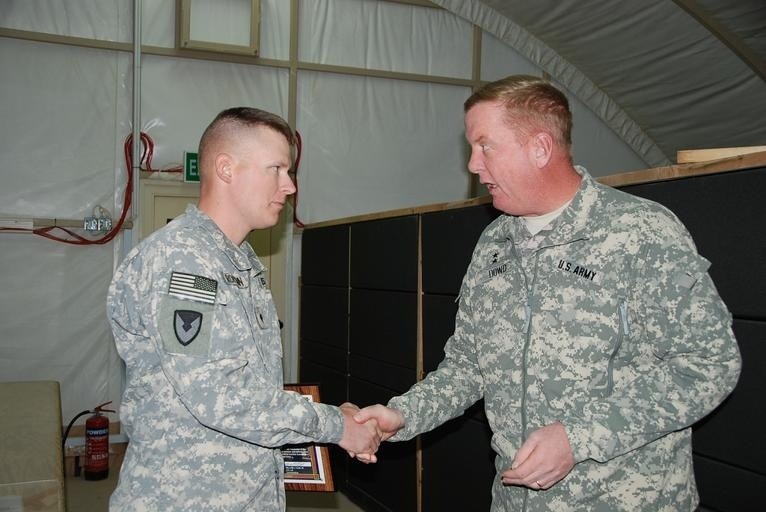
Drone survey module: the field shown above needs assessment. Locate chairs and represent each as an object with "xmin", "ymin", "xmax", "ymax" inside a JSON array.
[{"xmin": 0, "ymin": 380, "xmax": 67, "ymax": 512}]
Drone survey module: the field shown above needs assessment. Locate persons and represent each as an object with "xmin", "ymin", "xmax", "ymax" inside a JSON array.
[
  {"xmin": 352, "ymin": 74, "xmax": 743, "ymax": 512},
  {"xmin": 105, "ymin": 107, "xmax": 389, "ymax": 512}
]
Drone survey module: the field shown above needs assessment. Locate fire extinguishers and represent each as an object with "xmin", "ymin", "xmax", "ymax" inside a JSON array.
[{"xmin": 63, "ymin": 401, "xmax": 116, "ymax": 481}]
